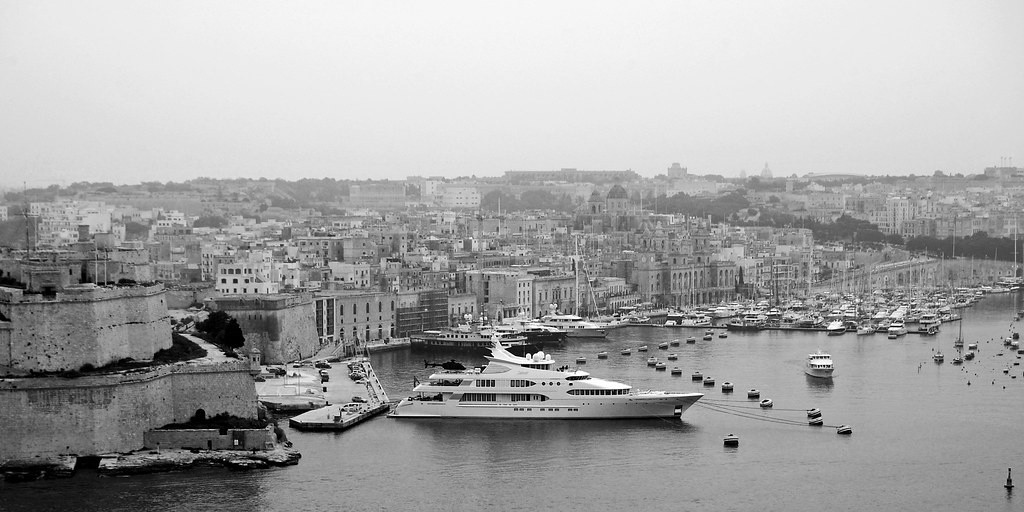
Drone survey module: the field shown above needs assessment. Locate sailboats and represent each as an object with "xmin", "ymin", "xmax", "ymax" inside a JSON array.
[
  {"xmin": 953, "ymin": 346, "xmax": 963, "ymax": 363},
  {"xmin": 955, "ymin": 316, "xmax": 964, "ymax": 347},
  {"xmin": 541, "ymin": 234, "xmax": 609, "ymax": 338}
]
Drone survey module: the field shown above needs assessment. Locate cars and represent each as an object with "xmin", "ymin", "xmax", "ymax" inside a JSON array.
[
  {"xmin": 352, "ymin": 396, "xmax": 368, "ymax": 403},
  {"xmin": 269, "ymin": 360, "xmax": 370, "ymax": 384}
]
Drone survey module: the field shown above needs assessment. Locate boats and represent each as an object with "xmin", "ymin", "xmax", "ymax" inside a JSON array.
[
  {"xmin": 385, "ymin": 341, "xmax": 704, "ymax": 420},
  {"xmin": 804, "ymin": 354, "xmax": 834, "ymax": 378},
  {"xmin": 969, "ymin": 343, "xmax": 977, "ymax": 348},
  {"xmin": 933, "ymin": 352, "xmax": 944, "ymax": 362},
  {"xmin": 964, "ymin": 353, "xmax": 975, "ymax": 360},
  {"xmin": 628, "ymin": 218, "xmax": 1024, "ymax": 333},
  {"xmin": 1003, "ymin": 333, "xmax": 1020, "ymax": 350},
  {"xmin": 856, "ymin": 325, "xmax": 873, "ymax": 336},
  {"xmin": 888, "ymin": 322, "xmax": 907, "ymax": 336},
  {"xmin": 428, "ymin": 300, "xmax": 574, "ymax": 354},
  {"xmin": 826, "ymin": 319, "xmax": 846, "ymax": 335}
]
